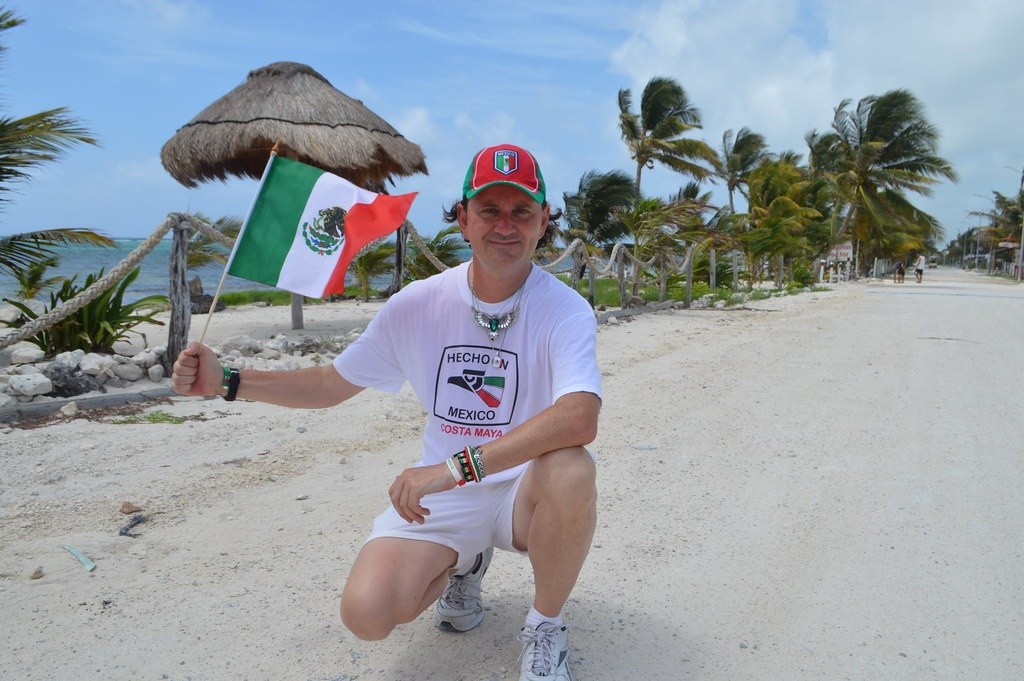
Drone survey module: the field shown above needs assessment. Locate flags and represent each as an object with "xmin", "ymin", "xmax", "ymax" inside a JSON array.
[{"xmin": 223, "ymin": 150, "xmax": 419, "ymax": 299}]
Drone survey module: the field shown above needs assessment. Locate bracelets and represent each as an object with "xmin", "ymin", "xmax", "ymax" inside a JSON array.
[
  {"xmin": 446, "ymin": 445, "xmax": 485, "ymax": 486},
  {"xmin": 219, "ymin": 366, "xmax": 240, "ymax": 402}
]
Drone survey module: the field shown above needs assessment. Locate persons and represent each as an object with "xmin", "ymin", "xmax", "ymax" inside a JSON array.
[
  {"xmin": 914, "ymin": 252, "xmax": 925, "ymax": 284},
  {"xmin": 171, "ymin": 145, "xmax": 603, "ymax": 681},
  {"xmin": 891, "ymin": 261, "xmax": 905, "ymax": 284}
]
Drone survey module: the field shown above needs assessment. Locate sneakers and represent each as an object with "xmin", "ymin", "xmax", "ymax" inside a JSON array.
[
  {"xmin": 516, "ymin": 622, "xmax": 572, "ymax": 681},
  {"xmin": 432, "ymin": 546, "xmax": 494, "ymax": 632}
]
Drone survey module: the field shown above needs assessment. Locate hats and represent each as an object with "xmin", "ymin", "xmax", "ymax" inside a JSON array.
[{"xmin": 463, "ymin": 144, "xmax": 546, "ymax": 204}]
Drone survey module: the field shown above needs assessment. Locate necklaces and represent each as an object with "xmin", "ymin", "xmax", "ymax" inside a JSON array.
[{"xmin": 469, "ymin": 264, "xmax": 529, "ymax": 368}]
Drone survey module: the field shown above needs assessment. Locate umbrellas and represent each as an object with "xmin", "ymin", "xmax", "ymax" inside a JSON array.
[{"xmin": 159, "ymin": 60, "xmax": 427, "ymax": 328}]
